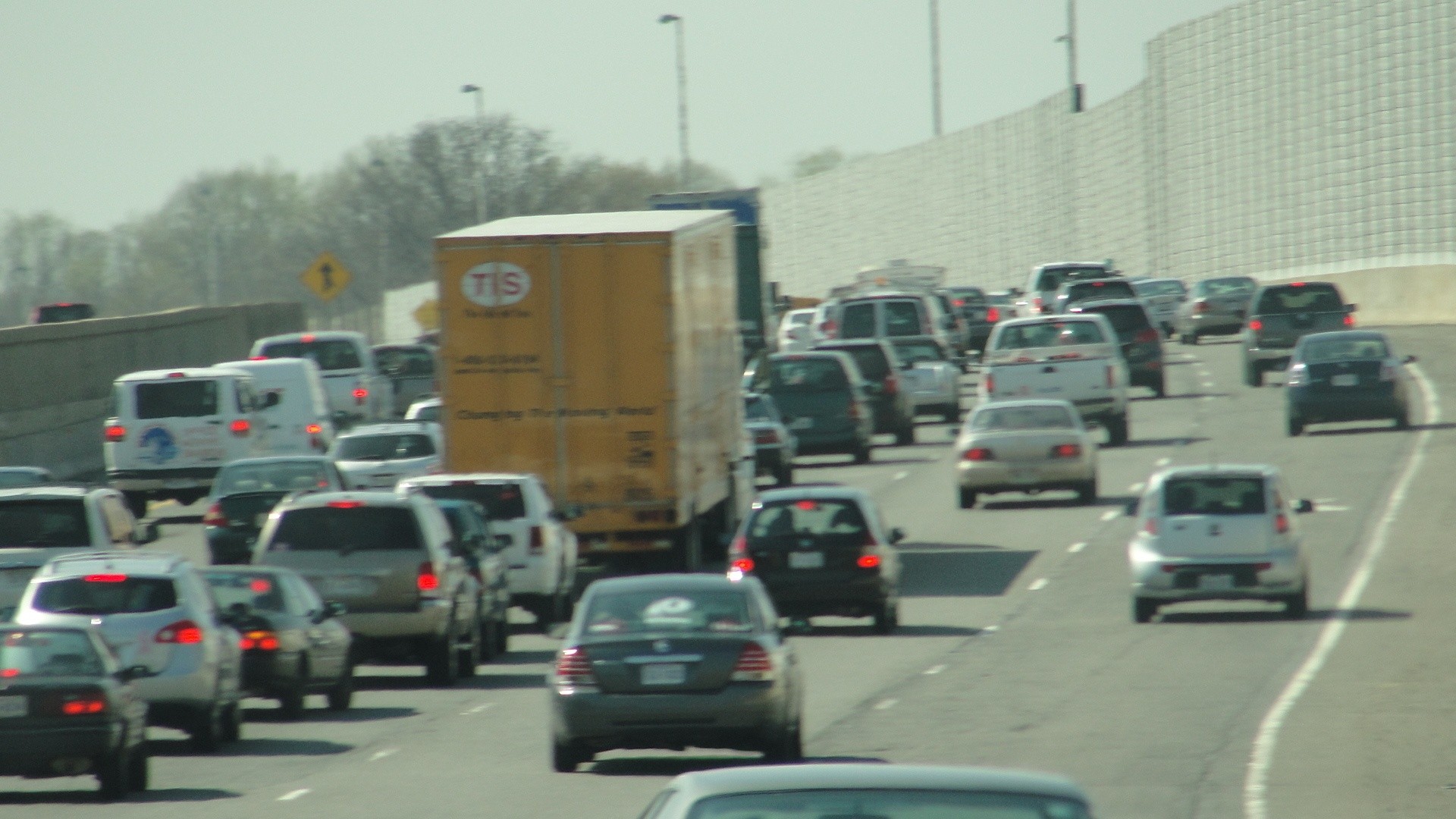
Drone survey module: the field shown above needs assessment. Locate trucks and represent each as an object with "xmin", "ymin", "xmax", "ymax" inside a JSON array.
[
  {"xmin": 644, "ymin": 186, "xmax": 772, "ymax": 363},
  {"xmin": 435, "ymin": 208, "xmax": 751, "ymax": 574}
]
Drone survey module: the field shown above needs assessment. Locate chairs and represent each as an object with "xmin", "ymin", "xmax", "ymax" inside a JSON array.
[
  {"xmin": 831, "ymin": 508, "xmax": 853, "ymax": 527},
  {"xmin": 768, "ymin": 517, "xmax": 790, "ymax": 540}
]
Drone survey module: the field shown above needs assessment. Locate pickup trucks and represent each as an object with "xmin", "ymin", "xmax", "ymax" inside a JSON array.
[{"xmin": 1241, "ymin": 282, "xmax": 1359, "ymax": 387}]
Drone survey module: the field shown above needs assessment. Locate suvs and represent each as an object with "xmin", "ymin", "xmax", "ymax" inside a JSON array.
[
  {"xmin": 979, "ymin": 314, "xmax": 1134, "ymax": 446},
  {"xmin": 250, "ymin": 332, "xmax": 396, "ymax": 429},
  {"xmin": 252, "ymin": 487, "xmax": 489, "ymax": 691},
  {"xmin": 321, "ymin": 417, "xmax": 446, "ymax": 494},
  {"xmin": 1, "ymin": 483, "xmax": 158, "ymax": 635},
  {"xmin": 396, "ymin": 472, "xmax": 584, "ymax": 628}
]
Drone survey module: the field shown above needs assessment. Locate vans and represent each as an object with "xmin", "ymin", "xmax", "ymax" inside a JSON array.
[
  {"xmin": 24, "ymin": 302, "xmax": 94, "ymax": 327},
  {"xmin": 208, "ymin": 356, "xmax": 347, "ymax": 456},
  {"xmin": 104, "ymin": 367, "xmax": 279, "ymax": 519}
]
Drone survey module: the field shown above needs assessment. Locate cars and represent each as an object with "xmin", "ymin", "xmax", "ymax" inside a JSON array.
[
  {"xmin": 204, "ymin": 452, "xmax": 342, "ymax": 566},
  {"xmin": 432, "ymin": 496, "xmax": 515, "ymax": 665},
  {"xmin": 1278, "ymin": 327, "xmax": 1418, "ymax": 437},
  {"xmin": 12, "ymin": 547, "xmax": 251, "ymax": 752},
  {"xmin": 1127, "ymin": 462, "xmax": 1314, "ymax": 626},
  {"xmin": 742, "ymin": 389, "xmax": 798, "ymax": 487},
  {"xmin": 202, "ymin": 561, "xmax": 356, "ymax": 723},
  {"xmin": 746, "ymin": 350, "xmax": 882, "ymax": 468},
  {"xmin": 638, "ymin": 760, "xmax": 1097, "ymax": 819},
  {"xmin": 366, "ymin": 327, "xmax": 447, "ymax": 429},
  {"xmin": 548, "ymin": 572, "xmax": 816, "ymax": 772},
  {"xmin": 1175, "ymin": 275, "xmax": 1258, "ymax": 343},
  {"xmin": 724, "ymin": 485, "xmax": 907, "ymax": 636},
  {"xmin": 765, "ymin": 256, "xmax": 1167, "ymax": 426},
  {"xmin": 1127, "ymin": 279, "xmax": 1188, "ymax": 337},
  {"xmin": 951, "ymin": 395, "xmax": 1098, "ymax": 511},
  {"xmin": 0, "ymin": 465, "xmax": 56, "ymax": 492},
  {"xmin": 0, "ymin": 622, "xmax": 153, "ymax": 802}
]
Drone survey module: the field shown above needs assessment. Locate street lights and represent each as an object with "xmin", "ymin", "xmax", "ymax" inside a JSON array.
[
  {"xmin": 460, "ymin": 82, "xmax": 489, "ymax": 227},
  {"xmin": 657, "ymin": 11, "xmax": 687, "ymax": 183}
]
